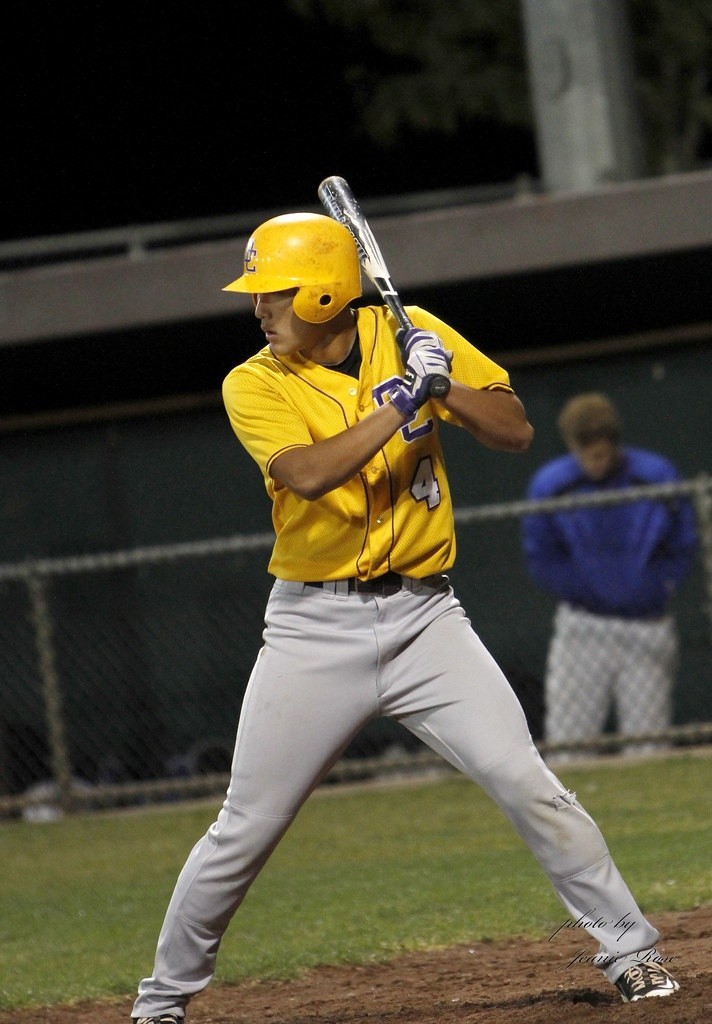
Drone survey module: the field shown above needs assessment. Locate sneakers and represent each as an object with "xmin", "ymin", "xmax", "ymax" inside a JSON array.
[{"xmin": 613, "ymin": 962, "xmax": 680, "ymax": 1001}]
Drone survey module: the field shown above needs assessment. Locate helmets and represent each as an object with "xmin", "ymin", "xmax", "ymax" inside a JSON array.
[{"xmin": 219, "ymin": 212, "xmax": 361, "ymax": 324}]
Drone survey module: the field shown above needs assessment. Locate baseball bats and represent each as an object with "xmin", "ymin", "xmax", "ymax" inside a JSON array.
[{"xmin": 317, "ymin": 175, "xmax": 451, "ymax": 398}]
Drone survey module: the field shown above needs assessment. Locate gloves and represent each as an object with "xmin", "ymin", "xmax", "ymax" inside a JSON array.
[
  {"xmin": 396, "ymin": 325, "xmax": 454, "ymax": 370},
  {"xmin": 388, "ymin": 345, "xmax": 453, "ymax": 415}
]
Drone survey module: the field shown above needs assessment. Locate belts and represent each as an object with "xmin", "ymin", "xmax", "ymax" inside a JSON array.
[{"xmin": 303, "ymin": 572, "xmax": 444, "ymax": 595}]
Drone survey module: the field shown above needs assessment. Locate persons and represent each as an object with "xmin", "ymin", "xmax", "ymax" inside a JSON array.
[
  {"xmin": 519, "ymin": 393, "xmax": 699, "ymax": 765},
  {"xmin": 131, "ymin": 211, "xmax": 679, "ymax": 1023}
]
